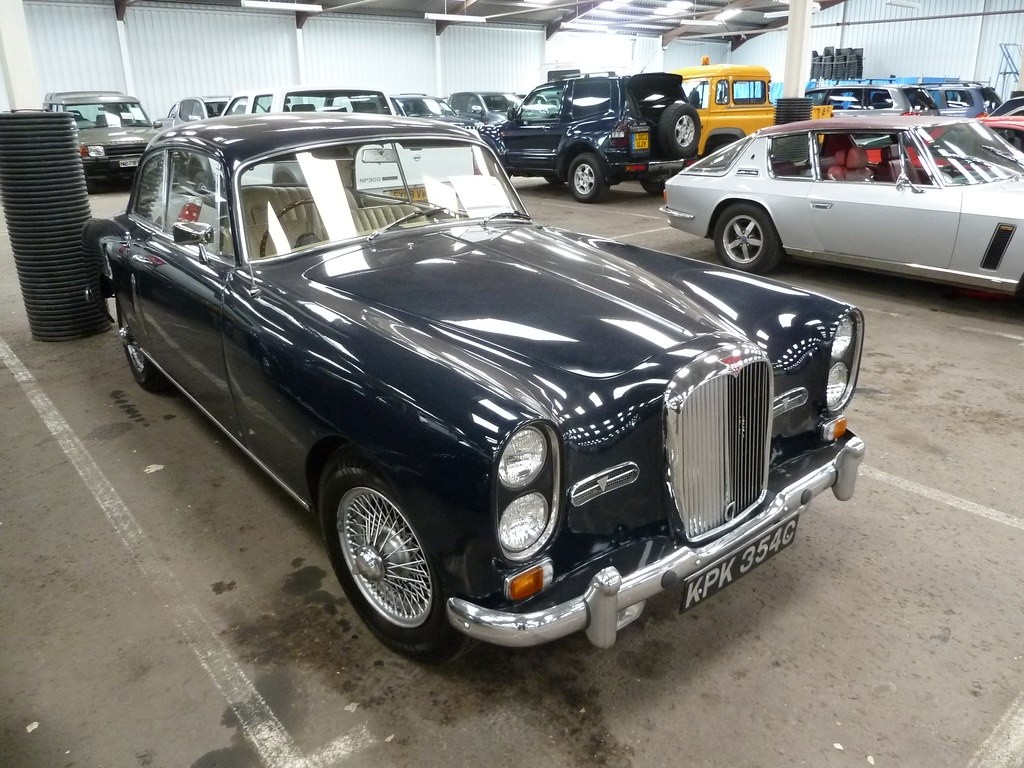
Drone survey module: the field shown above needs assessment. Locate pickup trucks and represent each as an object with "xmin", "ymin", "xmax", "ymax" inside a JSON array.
[{"xmin": 674, "ymin": 55, "xmax": 835, "ymax": 155}]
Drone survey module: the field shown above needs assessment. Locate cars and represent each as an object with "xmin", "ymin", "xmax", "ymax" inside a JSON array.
[
  {"xmin": 44, "ymin": 90, "xmax": 162, "ymax": 194},
  {"xmin": 158, "ymin": 96, "xmax": 230, "ymax": 131},
  {"xmin": 978, "ymin": 117, "xmax": 1024, "ymax": 152},
  {"xmin": 446, "ymin": 92, "xmax": 522, "ymax": 127},
  {"xmin": 101, "ymin": 111, "xmax": 868, "ymax": 663},
  {"xmin": 660, "ymin": 115, "xmax": 1024, "ymax": 319},
  {"xmin": 221, "ymin": 84, "xmax": 398, "ymax": 116}
]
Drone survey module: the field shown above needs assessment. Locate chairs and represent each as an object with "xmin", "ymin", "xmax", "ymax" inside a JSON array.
[
  {"xmin": 65, "ymin": 110, "xmax": 89, "ymax": 123},
  {"xmin": 238, "ymin": 182, "xmax": 428, "ymax": 258},
  {"xmin": 687, "ymin": 91, "xmax": 701, "ymax": 108},
  {"xmin": 775, "ymin": 143, "xmax": 922, "ymax": 184}
]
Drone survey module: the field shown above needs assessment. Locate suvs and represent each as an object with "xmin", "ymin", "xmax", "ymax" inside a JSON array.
[
  {"xmin": 390, "ymin": 93, "xmax": 485, "ymax": 131},
  {"xmin": 480, "ymin": 71, "xmax": 701, "ymax": 204},
  {"xmin": 909, "ymin": 81, "xmax": 1002, "ymax": 117},
  {"xmin": 806, "ymin": 79, "xmax": 941, "ymax": 116}
]
